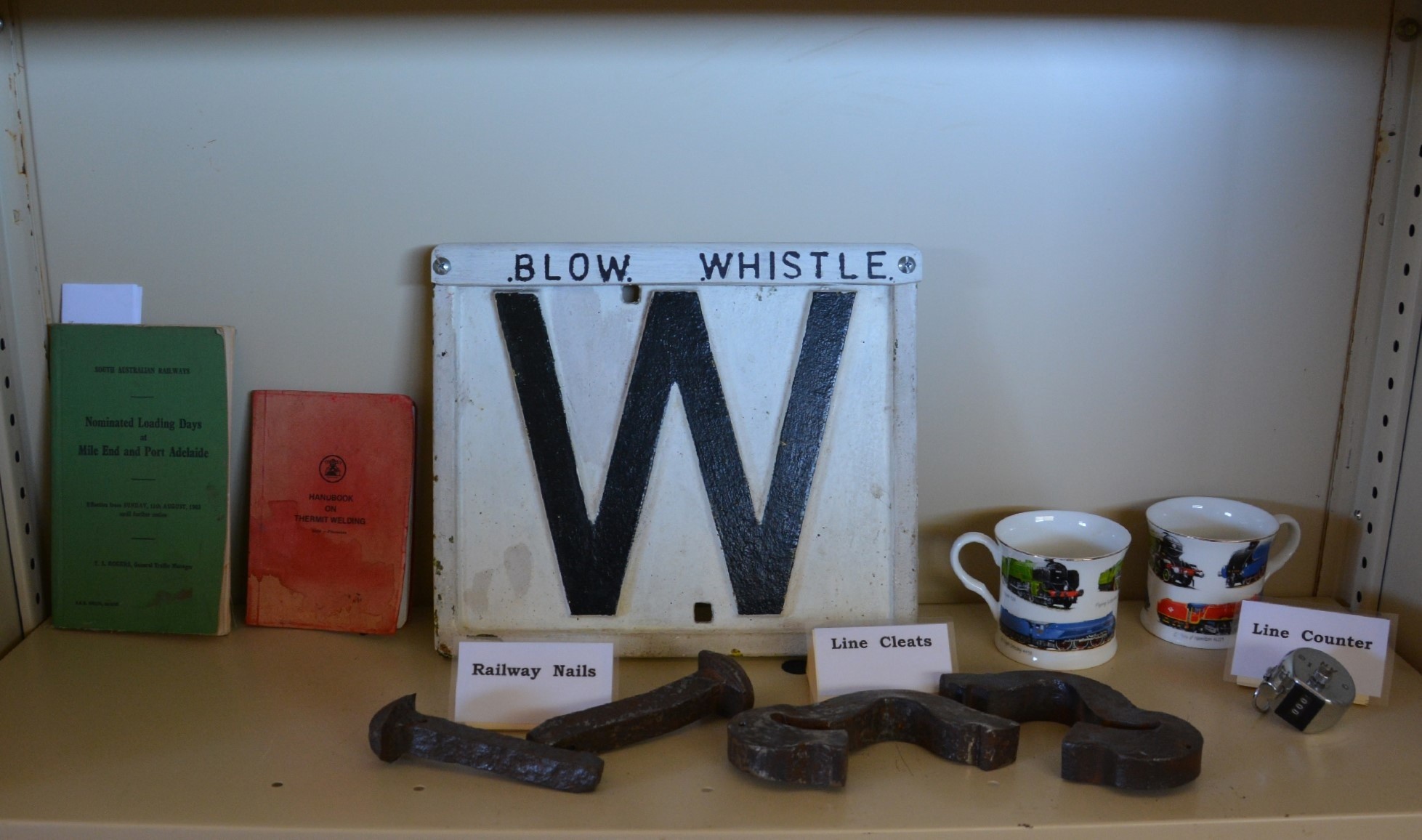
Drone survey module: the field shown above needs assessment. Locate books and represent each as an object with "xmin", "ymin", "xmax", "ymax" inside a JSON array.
[
  {"xmin": 238, "ymin": 391, "xmax": 417, "ymax": 636},
  {"xmin": 47, "ymin": 322, "xmax": 240, "ymax": 634}
]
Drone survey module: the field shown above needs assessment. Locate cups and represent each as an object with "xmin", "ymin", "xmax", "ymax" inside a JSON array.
[
  {"xmin": 949, "ymin": 509, "xmax": 1131, "ymax": 669},
  {"xmin": 1141, "ymin": 495, "xmax": 1302, "ymax": 647}
]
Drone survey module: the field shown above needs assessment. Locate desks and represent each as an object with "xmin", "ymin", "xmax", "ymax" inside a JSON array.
[{"xmin": 0, "ymin": 593, "xmax": 1422, "ymax": 840}]
